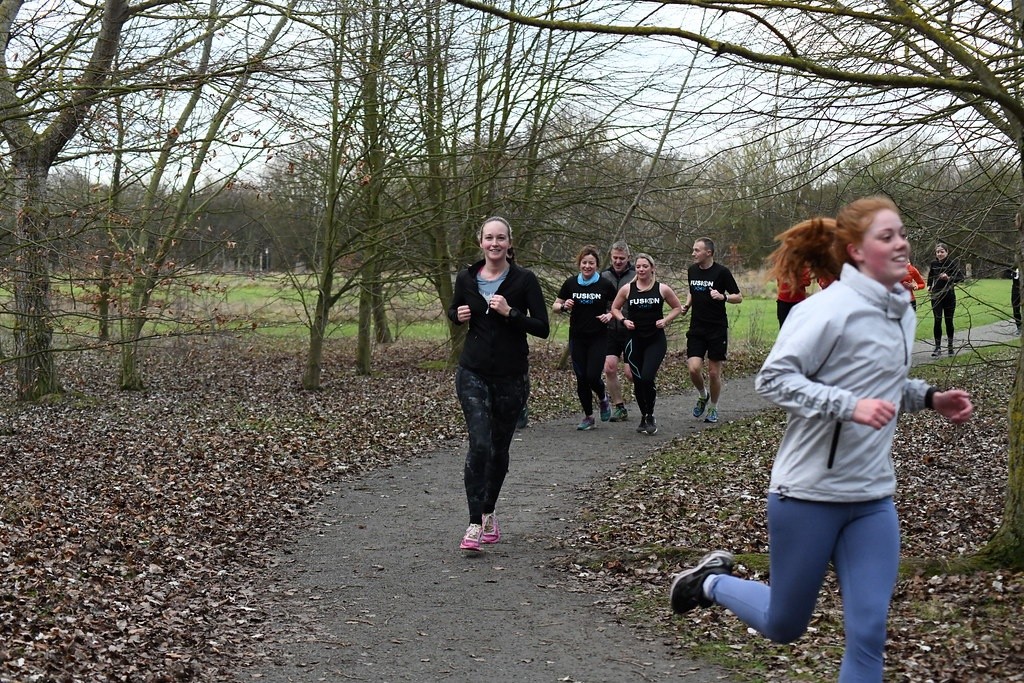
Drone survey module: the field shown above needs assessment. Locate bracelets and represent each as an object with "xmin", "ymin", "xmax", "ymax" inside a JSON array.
[
  {"xmin": 723, "ymin": 294, "xmax": 727, "ymax": 302},
  {"xmin": 726, "ymin": 295, "xmax": 728, "ymax": 301},
  {"xmin": 925, "ymin": 387, "xmax": 942, "ymax": 409},
  {"xmin": 620, "ymin": 317, "xmax": 627, "ymax": 324},
  {"xmin": 561, "ymin": 305, "xmax": 567, "ymax": 312}
]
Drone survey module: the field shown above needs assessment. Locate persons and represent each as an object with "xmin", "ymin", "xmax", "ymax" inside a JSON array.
[
  {"xmin": 599, "ymin": 243, "xmax": 636, "ymax": 423},
  {"xmin": 1011, "ymin": 253, "xmax": 1021, "ymax": 336},
  {"xmin": 776, "ymin": 268, "xmax": 812, "ymax": 329},
  {"xmin": 447, "ymin": 216, "xmax": 549, "ymax": 550},
  {"xmin": 899, "ymin": 258, "xmax": 925, "ymax": 311},
  {"xmin": 927, "ymin": 243, "xmax": 964, "ymax": 358},
  {"xmin": 681, "ymin": 237, "xmax": 742, "ymax": 423},
  {"xmin": 552, "ymin": 246, "xmax": 619, "ymax": 429},
  {"xmin": 612, "ymin": 253, "xmax": 681, "ymax": 433},
  {"xmin": 668, "ymin": 197, "xmax": 972, "ymax": 683}
]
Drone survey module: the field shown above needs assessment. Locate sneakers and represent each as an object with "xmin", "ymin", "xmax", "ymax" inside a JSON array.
[
  {"xmin": 703, "ymin": 404, "xmax": 719, "ymax": 423},
  {"xmin": 635, "ymin": 415, "xmax": 648, "ymax": 433},
  {"xmin": 459, "ymin": 523, "xmax": 484, "ymax": 550},
  {"xmin": 481, "ymin": 510, "xmax": 501, "ymax": 543},
  {"xmin": 608, "ymin": 407, "xmax": 629, "ymax": 422},
  {"xmin": 931, "ymin": 348, "xmax": 941, "ymax": 356},
  {"xmin": 646, "ymin": 414, "xmax": 658, "ymax": 436},
  {"xmin": 516, "ymin": 404, "xmax": 528, "ymax": 429},
  {"xmin": 632, "ymin": 383, "xmax": 636, "ymax": 395},
  {"xmin": 576, "ymin": 416, "xmax": 598, "ymax": 430},
  {"xmin": 947, "ymin": 349, "xmax": 955, "ymax": 357},
  {"xmin": 598, "ymin": 395, "xmax": 613, "ymax": 423},
  {"xmin": 692, "ymin": 391, "xmax": 711, "ymax": 418},
  {"xmin": 670, "ymin": 550, "xmax": 736, "ymax": 614}
]
